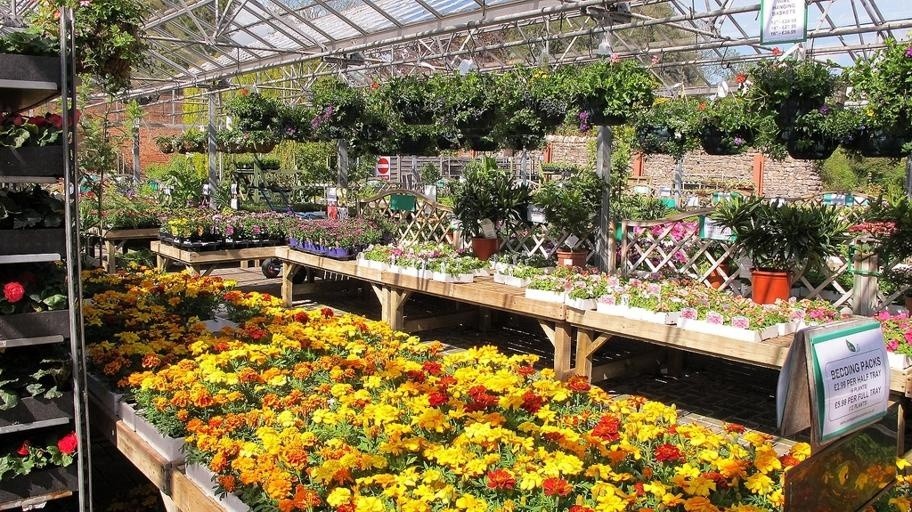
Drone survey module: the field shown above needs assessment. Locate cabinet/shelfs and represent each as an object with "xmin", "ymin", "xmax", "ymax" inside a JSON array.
[{"xmin": 0, "ymin": 1, "xmax": 97, "ymax": 512}]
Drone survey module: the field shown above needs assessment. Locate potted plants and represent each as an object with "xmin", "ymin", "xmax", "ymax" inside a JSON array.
[
  {"xmin": 711, "ymin": 190, "xmax": 858, "ymax": 306},
  {"xmin": 450, "ymin": 153, "xmax": 533, "ymax": 262},
  {"xmin": 531, "ymin": 161, "xmax": 613, "ymax": 269}
]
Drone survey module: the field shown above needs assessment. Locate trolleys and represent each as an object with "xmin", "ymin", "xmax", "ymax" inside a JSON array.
[{"xmin": 261, "ymin": 188, "xmax": 306, "ymax": 283}]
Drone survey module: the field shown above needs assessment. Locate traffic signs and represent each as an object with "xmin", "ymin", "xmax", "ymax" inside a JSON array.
[{"xmin": 374, "ymin": 156, "xmax": 391, "ymax": 181}]
